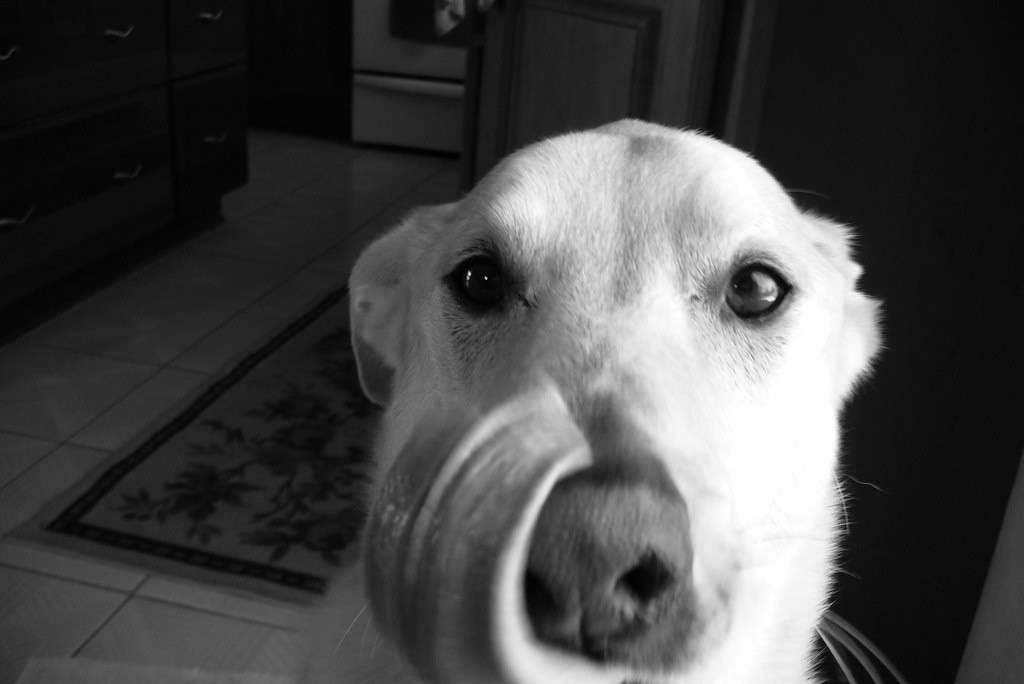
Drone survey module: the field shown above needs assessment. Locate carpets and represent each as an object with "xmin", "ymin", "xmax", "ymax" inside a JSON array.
[{"xmin": 0, "ymin": 277, "xmax": 384, "ymax": 610}]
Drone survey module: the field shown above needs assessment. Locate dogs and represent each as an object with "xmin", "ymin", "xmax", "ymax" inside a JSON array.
[{"xmin": 284, "ymin": 116, "xmax": 886, "ymax": 683}]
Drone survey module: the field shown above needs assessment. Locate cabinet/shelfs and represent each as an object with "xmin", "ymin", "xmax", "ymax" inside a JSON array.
[
  {"xmin": 0, "ymin": 0, "xmax": 178, "ymax": 347},
  {"xmin": 164, "ymin": 0, "xmax": 247, "ymax": 248}
]
